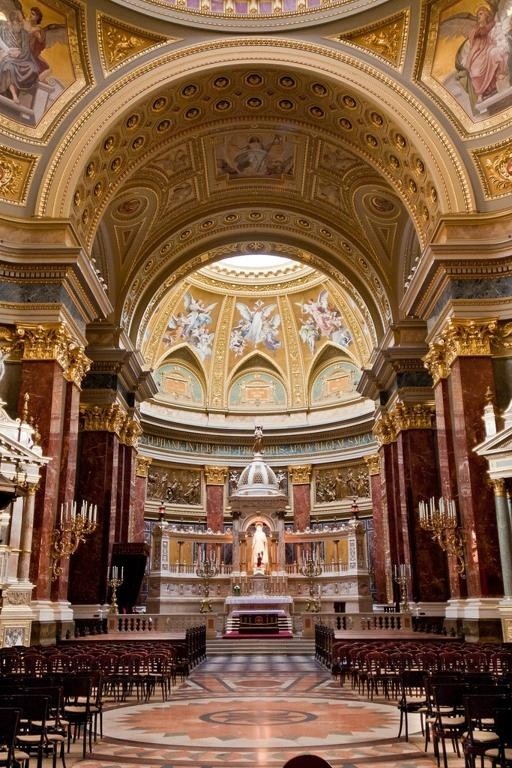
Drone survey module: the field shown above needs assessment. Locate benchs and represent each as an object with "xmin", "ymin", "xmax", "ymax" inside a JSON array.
[
  {"xmin": 313, "ymin": 623, "xmax": 461, "ymax": 675},
  {"xmin": 66, "ymin": 621, "xmax": 207, "ymax": 669}
]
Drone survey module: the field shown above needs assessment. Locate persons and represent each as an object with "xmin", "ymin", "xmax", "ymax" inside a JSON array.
[
  {"xmin": 20, "ymin": 6, "xmax": 50, "ymax": 76},
  {"xmin": 252, "ymin": 425, "xmax": 265, "ymax": 454},
  {"xmin": 460, "ymin": 6, "xmax": 510, "ymax": 103},
  {"xmin": 0, "ymin": 9, "xmax": 41, "ymax": 104},
  {"xmin": 160, "ymin": 289, "xmax": 351, "ymax": 357},
  {"xmin": 146, "ymin": 467, "xmax": 371, "ymax": 506},
  {"xmin": 251, "ymin": 523, "xmax": 271, "ymax": 570}
]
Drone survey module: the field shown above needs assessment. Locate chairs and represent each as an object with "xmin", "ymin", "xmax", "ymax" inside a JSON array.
[
  {"xmin": 323, "ymin": 643, "xmax": 512, "ymax": 768},
  {"xmin": 0, "ymin": 643, "xmax": 189, "ymax": 768}
]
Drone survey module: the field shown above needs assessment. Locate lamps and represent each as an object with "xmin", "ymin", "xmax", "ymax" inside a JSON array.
[
  {"xmin": 196, "ymin": 548, "xmax": 220, "ymax": 613},
  {"xmin": 49, "ymin": 497, "xmax": 98, "ymax": 583},
  {"xmin": 106, "ymin": 564, "xmax": 124, "ymax": 614},
  {"xmin": 418, "ymin": 497, "xmax": 465, "ymax": 574},
  {"xmin": 301, "ymin": 546, "xmax": 325, "ymax": 612},
  {"xmin": 394, "ymin": 562, "xmax": 411, "ymax": 612}
]
